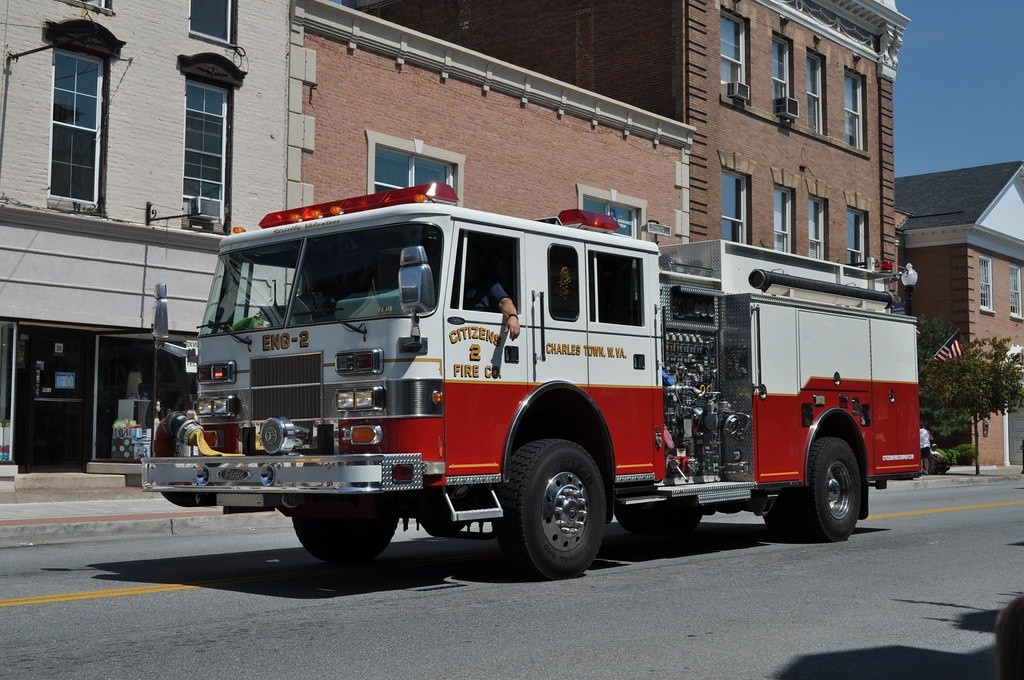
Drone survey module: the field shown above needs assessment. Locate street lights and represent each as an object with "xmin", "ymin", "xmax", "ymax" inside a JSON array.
[{"xmin": 901, "ymin": 263, "xmax": 917, "ymax": 315}]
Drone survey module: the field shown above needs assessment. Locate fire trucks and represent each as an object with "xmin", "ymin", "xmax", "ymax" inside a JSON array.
[{"xmin": 139, "ymin": 184, "xmax": 921, "ymax": 582}]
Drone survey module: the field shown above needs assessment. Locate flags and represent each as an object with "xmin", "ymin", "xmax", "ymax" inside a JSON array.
[{"xmin": 935, "ymin": 331, "xmax": 964, "ymax": 365}]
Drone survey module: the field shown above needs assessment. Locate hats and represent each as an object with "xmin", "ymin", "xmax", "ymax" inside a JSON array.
[{"xmin": 932, "ymin": 444, "xmax": 937, "ymax": 446}]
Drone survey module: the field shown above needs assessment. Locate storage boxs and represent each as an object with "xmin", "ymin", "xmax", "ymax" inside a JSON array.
[{"xmin": 112, "ymin": 428, "xmax": 156, "ymax": 459}]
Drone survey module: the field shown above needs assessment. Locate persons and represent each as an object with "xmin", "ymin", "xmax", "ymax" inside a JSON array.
[
  {"xmin": 126, "ymin": 371, "xmax": 143, "ymax": 400},
  {"xmin": 452, "ymin": 242, "xmax": 520, "ymax": 341},
  {"xmin": 919, "ymin": 420, "xmax": 950, "ymax": 475}
]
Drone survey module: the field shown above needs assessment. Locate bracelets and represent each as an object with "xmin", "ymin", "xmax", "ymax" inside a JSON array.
[{"xmin": 509, "ymin": 314, "xmax": 518, "ymax": 320}]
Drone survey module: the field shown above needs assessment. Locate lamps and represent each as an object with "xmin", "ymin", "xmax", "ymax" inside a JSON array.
[
  {"xmin": 780, "ymin": 15, "xmax": 791, "ymax": 26},
  {"xmin": 862, "ymin": 53, "xmax": 867, "ymax": 66},
  {"xmin": 815, "ymin": 34, "xmax": 822, "ymax": 44}
]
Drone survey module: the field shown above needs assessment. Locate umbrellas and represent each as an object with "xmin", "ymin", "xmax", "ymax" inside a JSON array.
[{"xmin": 152, "ymin": 283, "xmax": 169, "ymax": 341}]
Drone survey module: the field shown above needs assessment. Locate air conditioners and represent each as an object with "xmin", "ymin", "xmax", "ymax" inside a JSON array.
[
  {"xmin": 727, "ymin": 81, "xmax": 750, "ymax": 100},
  {"xmin": 776, "ymin": 97, "xmax": 800, "ymax": 118},
  {"xmin": 190, "ymin": 196, "xmax": 224, "ymax": 221}
]
